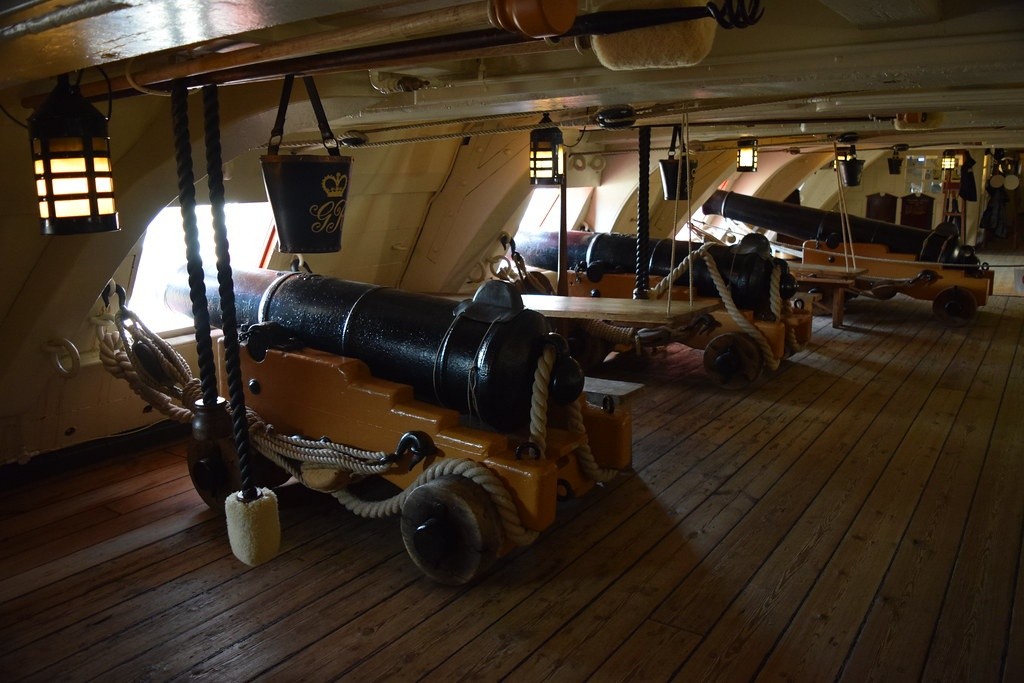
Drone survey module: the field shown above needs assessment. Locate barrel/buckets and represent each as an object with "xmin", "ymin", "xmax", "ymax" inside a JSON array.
[
  {"xmin": 259, "ymin": 156, "xmax": 352, "ymax": 255},
  {"xmin": 888, "ymin": 158, "xmax": 903, "ymax": 175},
  {"xmin": 662, "ymin": 159, "xmax": 698, "ymax": 201},
  {"xmin": 839, "ymin": 160, "xmax": 865, "ymax": 186}
]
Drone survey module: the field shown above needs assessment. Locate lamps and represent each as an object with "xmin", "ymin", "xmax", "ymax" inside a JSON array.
[
  {"xmin": 27, "ymin": 69, "xmax": 121, "ymax": 236},
  {"xmin": 737, "ymin": 139, "xmax": 759, "ymax": 172},
  {"xmin": 941, "ymin": 150, "xmax": 955, "ymax": 170},
  {"xmin": 834, "ymin": 146, "xmax": 852, "ymax": 171},
  {"xmin": 530, "ymin": 113, "xmax": 565, "ymax": 185}
]
